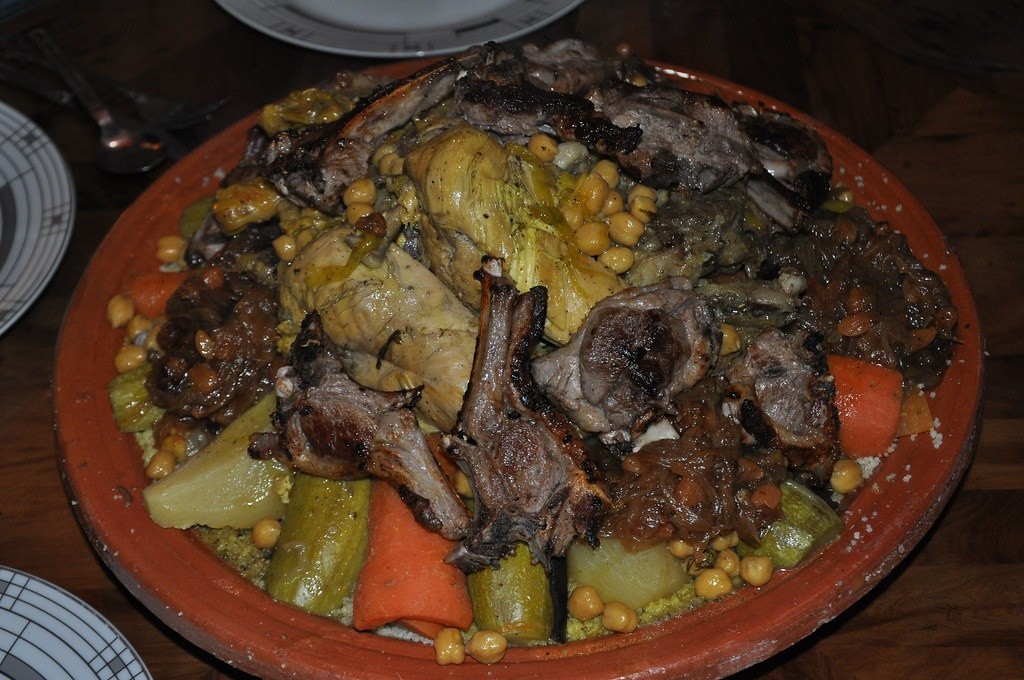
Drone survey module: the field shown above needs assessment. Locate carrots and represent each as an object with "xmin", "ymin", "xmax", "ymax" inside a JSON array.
[
  {"xmin": 822, "ymin": 351, "xmax": 904, "ymax": 460},
  {"xmin": 354, "ymin": 432, "xmax": 475, "ymax": 640},
  {"xmin": 131, "ymin": 264, "xmax": 223, "ymax": 317}
]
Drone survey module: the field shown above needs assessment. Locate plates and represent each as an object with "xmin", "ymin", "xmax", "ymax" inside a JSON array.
[
  {"xmin": 214, "ymin": 0, "xmax": 584, "ymax": 59},
  {"xmin": 0, "ymin": 100, "xmax": 76, "ymax": 337},
  {"xmin": 0, "ymin": 565, "xmax": 154, "ymax": 680}
]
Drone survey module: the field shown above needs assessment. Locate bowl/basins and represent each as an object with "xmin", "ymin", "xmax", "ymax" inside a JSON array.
[{"xmin": 51, "ymin": 52, "xmax": 988, "ymax": 680}]
